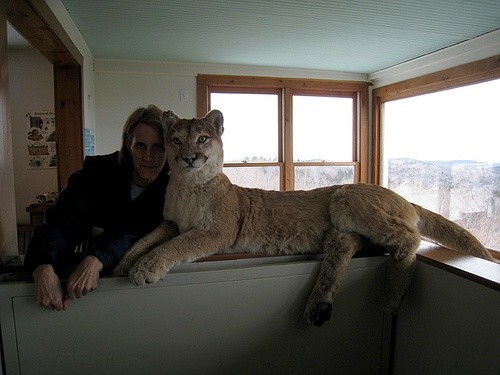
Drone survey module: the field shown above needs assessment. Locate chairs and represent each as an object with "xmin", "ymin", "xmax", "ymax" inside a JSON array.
[{"xmin": 17, "ymin": 224, "xmax": 34, "ymax": 255}]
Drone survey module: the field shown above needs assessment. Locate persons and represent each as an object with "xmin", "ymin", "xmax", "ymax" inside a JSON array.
[{"xmin": 23, "ymin": 104, "xmax": 171, "ymax": 311}]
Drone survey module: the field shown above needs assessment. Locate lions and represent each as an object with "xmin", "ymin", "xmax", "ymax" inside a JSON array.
[{"xmin": 112, "ymin": 109, "xmax": 496, "ymax": 328}]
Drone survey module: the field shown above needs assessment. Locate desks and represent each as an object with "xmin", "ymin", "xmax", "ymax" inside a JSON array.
[{"xmin": 26, "ymin": 204, "xmax": 53, "ymax": 227}]
